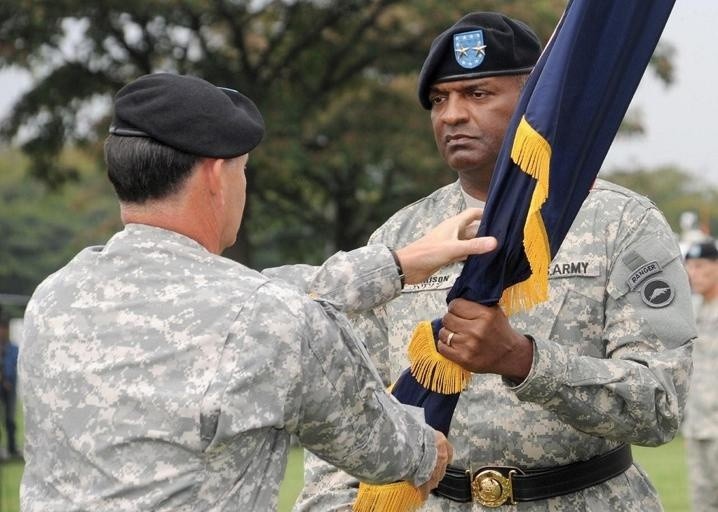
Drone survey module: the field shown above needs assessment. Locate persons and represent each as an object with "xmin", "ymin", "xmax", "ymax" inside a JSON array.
[
  {"xmin": 13, "ymin": 74, "xmax": 499, "ymax": 512},
  {"xmin": 682, "ymin": 238, "xmax": 718, "ymax": 512},
  {"xmin": 292, "ymin": 10, "xmax": 697, "ymax": 512},
  {"xmin": 1, "ymin": 316, "xmax": 24, "ymax": 462}
]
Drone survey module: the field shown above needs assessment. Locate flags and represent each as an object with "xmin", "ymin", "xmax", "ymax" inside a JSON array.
[{"xmin": 345, "ymin": 1, "xmax": 683, "ymax": 512}]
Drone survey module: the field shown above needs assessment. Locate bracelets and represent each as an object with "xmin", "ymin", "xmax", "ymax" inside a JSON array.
[{"xmin": 388, "ymin": 245, "xmax": 407, "ymax": 290}]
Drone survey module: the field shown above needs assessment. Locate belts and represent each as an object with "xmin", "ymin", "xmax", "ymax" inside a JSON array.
[{"xmin": 428, "ymin": 440, "xmax": 633, "ymax": 506}]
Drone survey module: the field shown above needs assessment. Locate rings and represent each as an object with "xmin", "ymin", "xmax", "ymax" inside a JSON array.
[{"xmin": 445, "ymin": 331, "xmax": 454, "ymax": 345}]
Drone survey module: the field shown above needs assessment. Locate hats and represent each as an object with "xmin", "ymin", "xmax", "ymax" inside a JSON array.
[
  {"xmin": 108, "ymin": 73, "xmax": 264, "ymax": 158},
  {"xmin": 418, "ymin": 11, "xmax": 543, "ymax": 110},
  {"xmin": 685, "ymin": 244, "xmax": 718, "ymax": 259}
]
